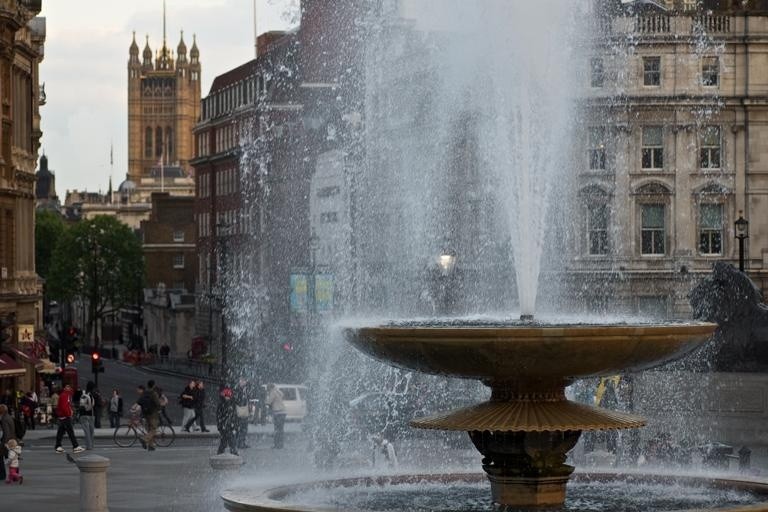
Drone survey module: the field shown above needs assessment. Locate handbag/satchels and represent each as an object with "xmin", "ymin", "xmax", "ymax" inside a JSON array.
[
  {"xmin": 237, "ymin": 405, "xmax": 249, "ymax": 418},
  {"xmin": 79, "ymin": 390, "xmax": 95, "ymax": 411}
]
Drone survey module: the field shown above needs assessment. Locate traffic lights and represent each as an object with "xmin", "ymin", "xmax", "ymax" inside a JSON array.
[
  {"xmin": 281, "ymin": 339, "xmax": 295, "ymax": 364},
  {"xmin": 65, "ymin": 324, "xmax": 75, "ymax": 363},
  {"xmin": 91, "ymin": 353, "xmax": 105, "ymax": 374}
]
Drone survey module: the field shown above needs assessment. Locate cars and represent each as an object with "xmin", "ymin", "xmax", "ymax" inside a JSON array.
[
  {"xmin": 237, "ymin": 381, "xmax": 311, "ymax": 420},
  {"xmin": 298, "ymin": 391, "xmax": 410, "ymax": 444}
]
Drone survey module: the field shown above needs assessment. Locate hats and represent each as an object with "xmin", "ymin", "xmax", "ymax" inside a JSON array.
[{"xmin": 6, "ymin": 439, "xmax": 16, "ymax": 449}]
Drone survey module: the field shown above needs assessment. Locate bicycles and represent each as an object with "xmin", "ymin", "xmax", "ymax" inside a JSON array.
[{"xmin": 113, "ymin": 409, "xmax": 179, "ymax": 449}]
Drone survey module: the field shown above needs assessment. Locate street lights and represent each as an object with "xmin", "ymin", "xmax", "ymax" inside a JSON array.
[
  {"xmin": 435, "ymin": 247, "xmax": 460, "ymax": 450},
  {"xmin": 215, "ymin": 213, "xmax": 233, "ymax": 427},
  {"xmin": 734, "ymin": 214, "xmax": 750, "ymax": 273}
]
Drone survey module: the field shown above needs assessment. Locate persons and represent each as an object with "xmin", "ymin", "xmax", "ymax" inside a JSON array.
[
  {"xmin": 5, "ymin": 440, "xmax": 23, "ymax": 485},
  {"xmin": 159, "ymin": 339, "xmax": 170, "ymax": 370},
  {"xmin": 368, "ymin": 428, "xmax": 396, "ymax": 471},
  {"xmin": 0, "ymin": 375, "xmax": 289, "ymax": 455},
  {"xmin": 584, "ymin": 428, "xmax": 692, "ymax": 471},
  {"xmin": 0, "ymin": 404, "xmax": 18, "ymax": 482}
]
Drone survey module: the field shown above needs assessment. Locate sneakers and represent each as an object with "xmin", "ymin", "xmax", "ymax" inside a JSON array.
[
  {"xmin": 182, "ymin": 425, "xmax": 209, "ymax": 432},
  {"xmin": 56, "ymin": 447, "xmax": 64, "ymax": 453},
  {"xmin": 138, "ymin": 438, "xmax": 155, "ymax": 451},
  {"xmin": 73, "ymin": 446, "xmax": 84, "ymax": 454},
  {"xmin": 6, "ymin": 476, "xmax": 23, "ymax": 485}
]
